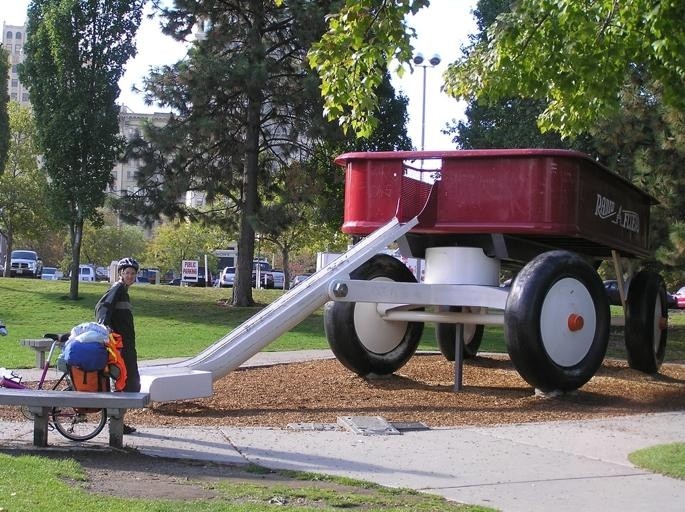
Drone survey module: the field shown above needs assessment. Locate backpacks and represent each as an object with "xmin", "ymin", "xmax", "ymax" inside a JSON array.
[{"xmin": 95, "ymin": 292, "xmax": 108, "ymax": 322}]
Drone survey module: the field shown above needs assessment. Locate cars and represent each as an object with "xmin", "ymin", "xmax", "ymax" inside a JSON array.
[
  {"xmin": 666, "ymin": 287, "xmax": 685, "ymax": 308},
  {"xmin": 602, "ymin": 280, "xmax": 619, "ymax": 305}
]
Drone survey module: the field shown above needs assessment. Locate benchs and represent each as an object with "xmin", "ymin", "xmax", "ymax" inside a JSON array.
[
  {"xmin": 21, "ymin": 337, "xmax": 58, "ymax": 368},
  {"xmin": 0, "ymin": 386, "xmax": 151, "ymax": 449}
]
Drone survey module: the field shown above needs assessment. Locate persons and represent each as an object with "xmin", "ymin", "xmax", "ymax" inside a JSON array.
[
  {"xmin": 94, "ymin": 258, "xmax": 141, "ymax": 434},
  {"xmin": 35, "ymin": 258, "xmax": 43, "ymax": 279}
]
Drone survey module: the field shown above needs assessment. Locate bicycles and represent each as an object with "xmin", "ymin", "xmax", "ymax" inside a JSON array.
[{"xmin": 0, "ymin": 333, "xmax": 107, "ymax": 441}]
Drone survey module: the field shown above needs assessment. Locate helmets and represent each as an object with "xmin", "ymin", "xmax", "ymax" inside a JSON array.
[{"xmin": 117, "ymin": 258, "xmax": 138, "ymax": 271}]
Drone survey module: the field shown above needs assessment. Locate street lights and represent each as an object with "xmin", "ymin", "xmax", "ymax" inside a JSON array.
[{"xmin": 413, "ymin": 50, "xmax": 441, "ymax": 150}]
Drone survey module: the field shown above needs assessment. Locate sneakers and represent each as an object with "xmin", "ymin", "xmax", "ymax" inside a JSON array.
[{"xmin": 123, "ymin": 425, "xmax": 136, "ymax": 434}]
